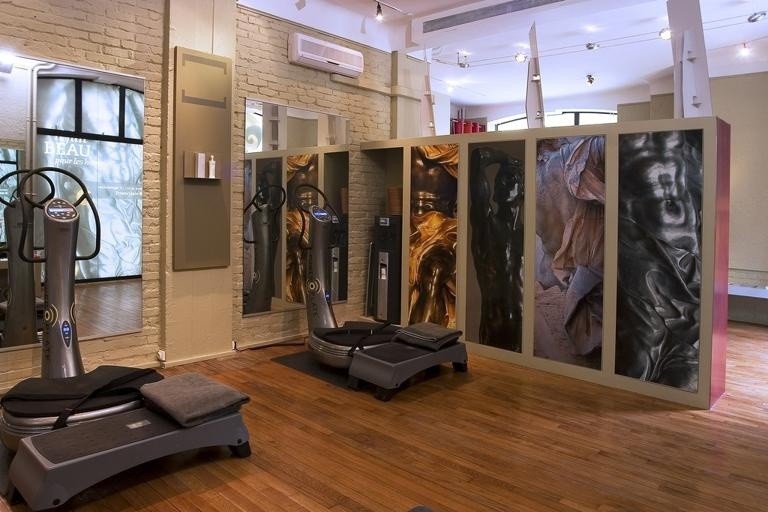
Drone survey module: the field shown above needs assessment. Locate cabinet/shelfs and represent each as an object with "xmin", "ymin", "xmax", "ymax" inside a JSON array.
[{"xmin": 364, "ymin": 115, "xmax": 730, "ymax": 410}]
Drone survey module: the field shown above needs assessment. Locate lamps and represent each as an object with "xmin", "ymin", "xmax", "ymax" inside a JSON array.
[
  {"xmin": 376, "ymin": 2, "xmax": 383, "ymax": 22},
  {"xmin": 739, "ymin": 41, "xmax": 751, "ymax": 57}
]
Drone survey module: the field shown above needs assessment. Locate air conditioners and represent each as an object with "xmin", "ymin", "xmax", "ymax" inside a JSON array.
[{"xmin": 288, "ymin": 32, "xmax": 363, "ymax": 79}]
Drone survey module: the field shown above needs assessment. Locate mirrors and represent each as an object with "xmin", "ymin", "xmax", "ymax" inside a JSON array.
[
  {"xmin": 243, "ymin": 97, "xmax": 347, "ymax": 321},
  {"xmin": 0, "ymin": 52, "xmax": 143, "ymax": 352}
]
